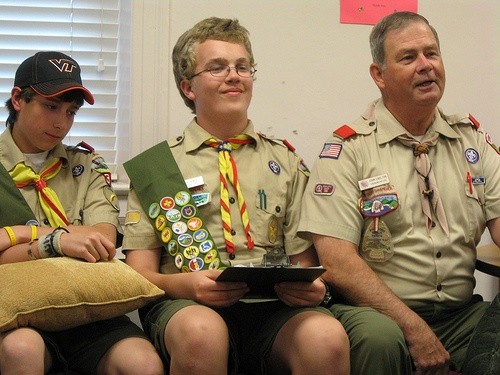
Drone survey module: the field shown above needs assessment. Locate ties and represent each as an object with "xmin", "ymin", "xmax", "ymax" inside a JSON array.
[
  {"xmin": 396, "ymin": 132, "xmax": 449, "ymax": 236},
  {"xmin": 205, "ymin": 135, "xmax": 255, "ymax": 254},
  {"xmin": 7, "ymin": 155, "xmax": 69, "ymax": 233}
]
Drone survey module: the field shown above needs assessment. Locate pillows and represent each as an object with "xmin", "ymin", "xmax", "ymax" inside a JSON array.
[{"xmin": 0, "ymin": 257, "xmax": 166, "ymax": 334}]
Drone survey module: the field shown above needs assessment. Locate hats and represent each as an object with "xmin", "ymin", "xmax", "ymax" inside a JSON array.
[{"xmin": 14, "ymin": 52, "xmax": 94, "ymax": 106}]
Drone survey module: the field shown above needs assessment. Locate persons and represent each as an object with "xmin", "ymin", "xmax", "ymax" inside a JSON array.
[
  {"xmin": 0, "ymin": 51, "xmax": 164, "ymax": 375},
  {"xmin": 295, "ymin": 10, "xmax": 500, "ymax": 375},
  {"xmin": 121, "ymin": 16, "xmax": 351, "ymax": 375}
]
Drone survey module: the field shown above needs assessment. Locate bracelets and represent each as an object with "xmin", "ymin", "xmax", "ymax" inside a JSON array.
[
  {"xmin": 3, "ymin": 225, "xmax": 17, "ymax": 246},
  {"xmin": 27, "ymin": 225, "xmax": 70, "ymax": 260},
  {"xmin": 29, "ymin": 225, "xmax": 38, "ymax": 242}
]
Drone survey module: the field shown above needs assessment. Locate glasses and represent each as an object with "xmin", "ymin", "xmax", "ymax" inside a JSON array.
[{"xmin": 188, "ymin": 65, "xmax": 257, "ymax": 80}]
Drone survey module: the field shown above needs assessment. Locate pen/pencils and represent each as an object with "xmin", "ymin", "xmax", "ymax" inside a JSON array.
[
  {"xmin": 258, "ymin": 187, "xmax": 267, "ymax": 209},
  {"xmin": 466, "ymin": 171, "xmax": 472, "ymax": 194}
]
